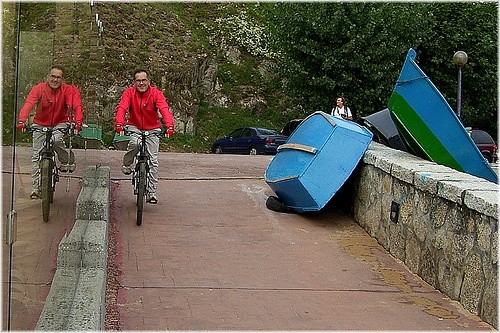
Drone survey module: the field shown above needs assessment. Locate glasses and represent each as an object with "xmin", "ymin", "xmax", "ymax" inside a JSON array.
[
  {"xmin": 50, "ymin": 74, "xmax": 62, "ymax": 80},
  {"xmin": 134, "ymin": 79, "xmax": 149, "ymax": 83}
]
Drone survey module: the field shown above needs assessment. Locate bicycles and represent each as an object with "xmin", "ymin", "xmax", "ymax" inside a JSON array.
[
  {"xmin": 117, "ymin": 125, "xmax": 174, "ymax": 227},
  {"xmin": 17, "ymin": 123, "xmax": 77, "ymax": 222}
]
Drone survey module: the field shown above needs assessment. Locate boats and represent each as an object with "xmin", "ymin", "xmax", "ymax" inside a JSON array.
[
  {"xmin": 263, "ymin": 110, "xmax": 374, "ymax": 212},
  {"xmin": 386, "ymin": 48, "xmax": 499, "ymax": 185}
]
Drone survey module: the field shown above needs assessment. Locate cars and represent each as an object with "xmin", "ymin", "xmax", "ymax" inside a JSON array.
[
  {"xmin": 212, "ymin": 126, "xmax": 287, "ymax": 156},
  {"xmin": 469, "ymin": 129, "xmax": 497, "ymax": 164}
]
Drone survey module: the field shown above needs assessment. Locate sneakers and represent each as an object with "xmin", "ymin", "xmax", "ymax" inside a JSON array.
[
  {"xmin": 30, "ymin": 188, "xmax": 41, "ymax": 198},
  {"xmin": 60, "ymin": 162, "xmax": 69, "ymax": 173},
  {"xmin": 122, "ymin": 164, "xmax": 132, "ymax": 175},
  {"xmin": 69, "ymin": 162, "xmax": 77, "ymax": 172},
  {"xmin": 148, "ymin": 193, "xmax": 158, "ymax": 204}
]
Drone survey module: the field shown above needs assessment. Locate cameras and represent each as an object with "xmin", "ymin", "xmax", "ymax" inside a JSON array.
[{"xmin": 341, "ymin": 114, "xmax": 346, "ymax": 117}]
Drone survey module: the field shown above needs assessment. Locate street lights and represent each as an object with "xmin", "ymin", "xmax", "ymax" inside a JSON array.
[{"xmin": 453, "ymin": 51, "xmax": 468, "ymax": 117}]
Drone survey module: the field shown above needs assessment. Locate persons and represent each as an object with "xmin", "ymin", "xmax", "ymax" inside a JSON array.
[
  {"xmin": 330, "ymin": 96, "xmax": 353, "ymax": 121},
  {"xmin": 114, "ymin": 69, "xmax": 175, "ymax": 205},
  {"xmin": 16, "ymin": 65, "xmax": 83, "ymax": 200}
]
